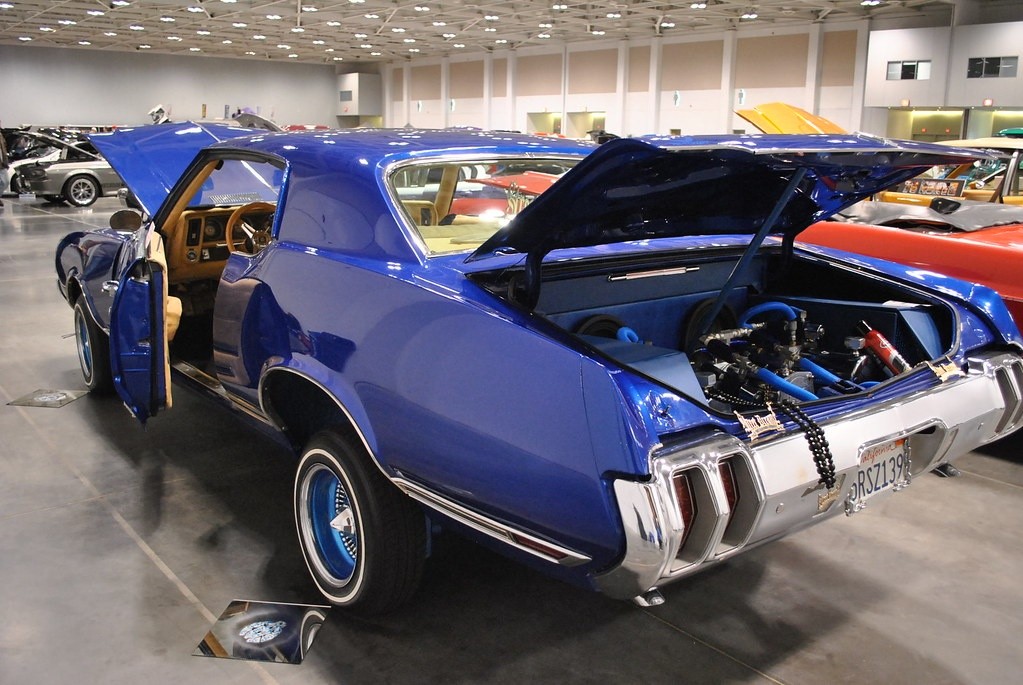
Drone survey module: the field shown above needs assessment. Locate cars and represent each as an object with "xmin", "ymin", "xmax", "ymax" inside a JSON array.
[
  {"xmin": 732, "ymin": 103, "xmax": 1023, "ymax": 339},
  {"xmin": 0, "ymin": 123, "xmax": 126, "ymax": 210}
]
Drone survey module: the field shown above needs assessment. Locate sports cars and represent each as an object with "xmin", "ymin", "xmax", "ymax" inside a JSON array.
[{"xmin": 57, "ymin": 119, "xmax": 1023, "ymax": 633}]
